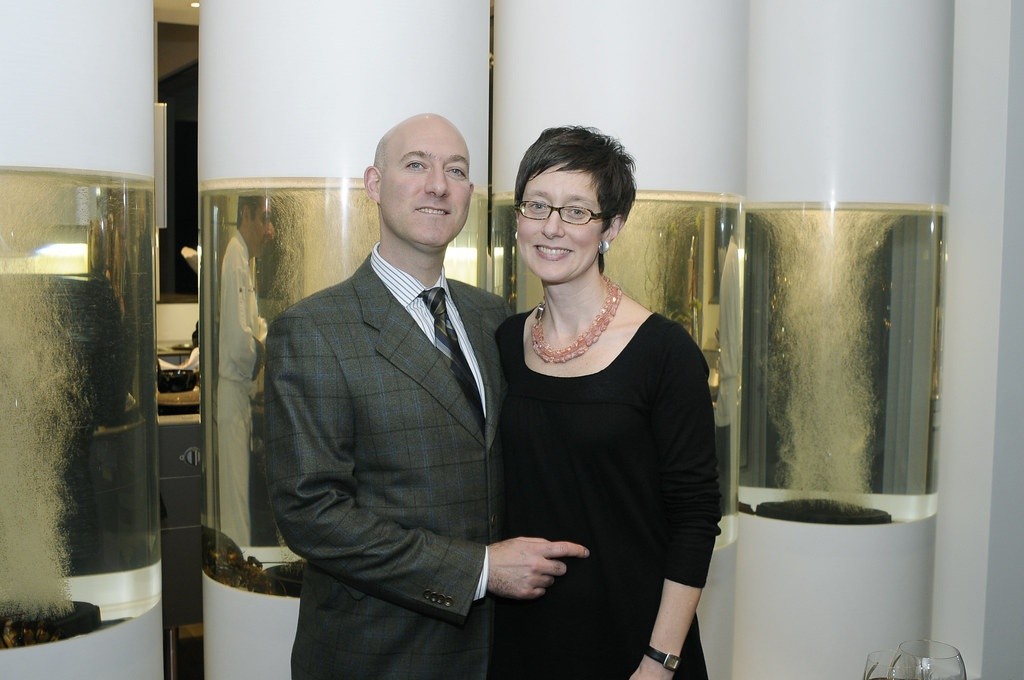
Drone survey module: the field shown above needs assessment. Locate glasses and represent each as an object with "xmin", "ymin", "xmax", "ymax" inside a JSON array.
[{"xmin": 514, "ymin": 199, "xmax": 612, "ymax": 226}]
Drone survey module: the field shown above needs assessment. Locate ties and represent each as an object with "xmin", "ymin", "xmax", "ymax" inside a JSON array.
[{"xmin": 420, "ymin": 287, "xmax": 485, "ymax": 443}]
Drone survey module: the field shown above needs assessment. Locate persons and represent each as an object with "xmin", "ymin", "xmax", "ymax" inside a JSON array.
[
  {"xmin": 487, "ymin": 125, "xmax": 723, "ymax": 680},
  {"xmin": 267, "ymin": 113, "xmax": 591, "ymax": 680},
  {"xmin": 217, "ymin": 195, "xmax": 282, "ymax": 548}
]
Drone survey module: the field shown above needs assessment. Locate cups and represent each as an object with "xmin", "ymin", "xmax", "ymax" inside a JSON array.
[
  {"xmin": 863, "ymin": 651, "xmax": 917, "ymax": 680},
  {"xmin": 887, "ymin": 640, "xmax": 967, "ymax": 680}
]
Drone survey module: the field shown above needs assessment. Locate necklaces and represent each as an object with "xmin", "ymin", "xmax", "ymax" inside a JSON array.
[{"xmin": 531, "ymin": 277, "xmax": 622, "ymax": 363}]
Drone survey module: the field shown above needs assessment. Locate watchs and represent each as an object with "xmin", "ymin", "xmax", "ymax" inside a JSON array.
[{"xmin": 644, "ymin": 646, "xmax": 680, "ymax": 671}]
